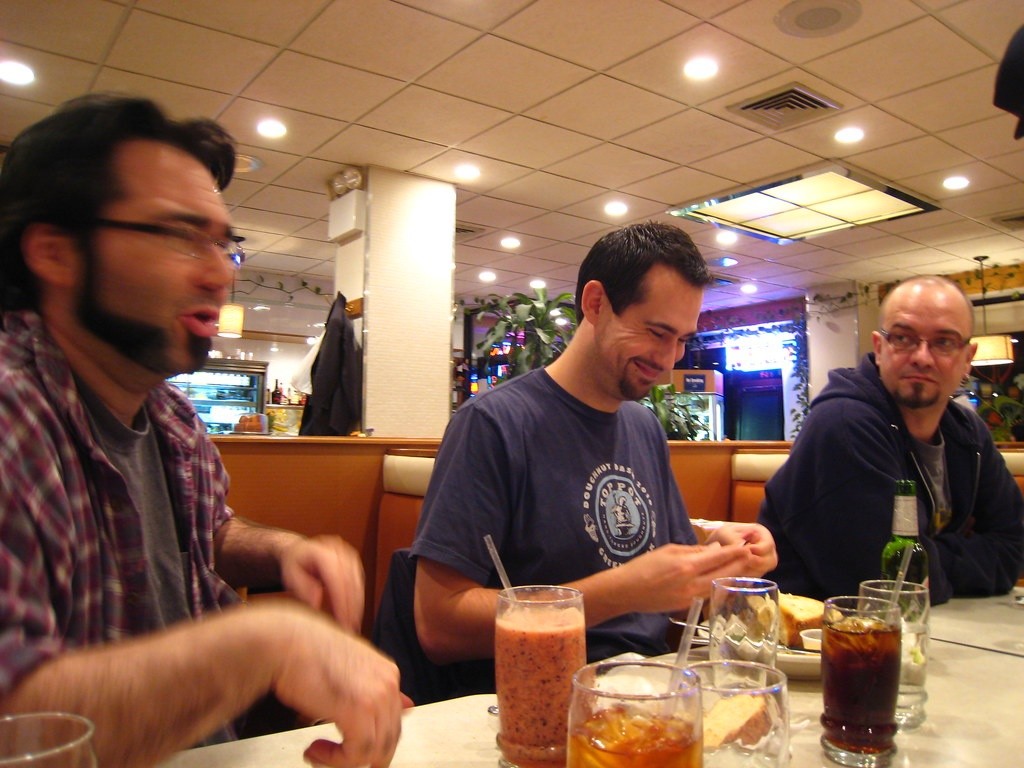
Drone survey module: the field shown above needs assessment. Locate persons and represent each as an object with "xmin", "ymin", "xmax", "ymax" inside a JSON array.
[
  {"xmin": 406, "ymin": 220, "xmax": 778, "ymax": 694},
  {"xmin": 0, "ymin": 93, "xmax": 413, "ymax": 768},
  {"xmin": 757, "ymin": 273, "xmax": 1024, "ymax": 616}
]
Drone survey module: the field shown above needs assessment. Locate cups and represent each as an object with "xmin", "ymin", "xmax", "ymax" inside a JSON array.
[
  {"xmin": 0, "ymin": 711, "xmax": 99, "ymax": 768},
  {"xmin": 819, "ymin": 595, "xmax": 902, "ymax": 768},
  {"xmin": 708, "ymin": 576, "xmax": 780, "ymax": 698},
  {"xmin": 494, "ymin": 585, "xmax": 588, "ymax": 768},
  {"xmin": 857, "ymin": 579, "xmax": 930, "ymax": 733},
  {"xmin": 798, "ymin": 629, "xmax": 821, "ymax": 653},
  {"xmin": 565, "ymin": 659, "xmax": 704, "ymax": 768},
  {"xmin": 687, "ymin": 659, "xmax": 792, "ymax": 768}
]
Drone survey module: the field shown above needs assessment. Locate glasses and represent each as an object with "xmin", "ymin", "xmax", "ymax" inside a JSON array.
[
  {"xmin": 879, "ymin": 327, "xmax": 971, "ymax": 356},
  {"xmin": 96, "ymin": 218, "xmax": 246, "ymax": 271}
]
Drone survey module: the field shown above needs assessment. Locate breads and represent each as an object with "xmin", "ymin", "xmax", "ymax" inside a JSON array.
[
  {"xmin": 755, "ymin": 591, "xmax": 845, "ymax": 650},
  {"xmin": 233, "ymin": 413, "xmax": 263, "ymax": 433},
  {"xmin": 704, "ymin": 693, "xmax": 775, "ymax": 754}
]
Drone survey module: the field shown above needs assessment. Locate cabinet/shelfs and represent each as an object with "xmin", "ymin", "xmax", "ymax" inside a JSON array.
[{"xmin": 167, "ymin": 358, "xmax": 268, "ymax": 435}]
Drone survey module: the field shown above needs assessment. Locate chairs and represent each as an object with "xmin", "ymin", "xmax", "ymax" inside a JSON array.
[
  {"xmin": 732, "ymin": 448, "xmax": 793, "ymax": 523},
  {"xmin": 372, "ymin": 448, "xmax": 442, "ymax": 622},
  {"xmin": 997, "ymin": 448, "xmax": 1024, "ymax": 499}
]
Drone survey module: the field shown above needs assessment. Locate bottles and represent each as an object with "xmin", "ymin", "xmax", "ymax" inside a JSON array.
[{"xmin": 874, "ymin": 479, "xmax": 928, "ymax": 666}]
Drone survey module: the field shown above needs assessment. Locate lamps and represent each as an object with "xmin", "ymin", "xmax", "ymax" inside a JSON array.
[
  {"xmin": 215, "ymin": 235, "xmax": 247, "ymax": 340},
  {"xmin": 969, "ymin": 255, "xmax": 1014, "ymax": 367},
  {"xmin": 326, "ymin": 166, "xmax": 366, "ymax": 242}
]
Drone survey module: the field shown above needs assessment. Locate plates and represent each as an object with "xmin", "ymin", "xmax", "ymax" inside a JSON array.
[{"xmin": 698, "ymin": 618, "xmax": 822, "ymax": 682}]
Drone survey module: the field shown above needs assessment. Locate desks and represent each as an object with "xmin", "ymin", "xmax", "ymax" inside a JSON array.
[
  {"xmin": 165, "ymin": 643, "xmax": 1024, "ymax": 768},
  {"xmin": 926, "ymin": 593, "xmax": 1024, "ymax": 656}
]
